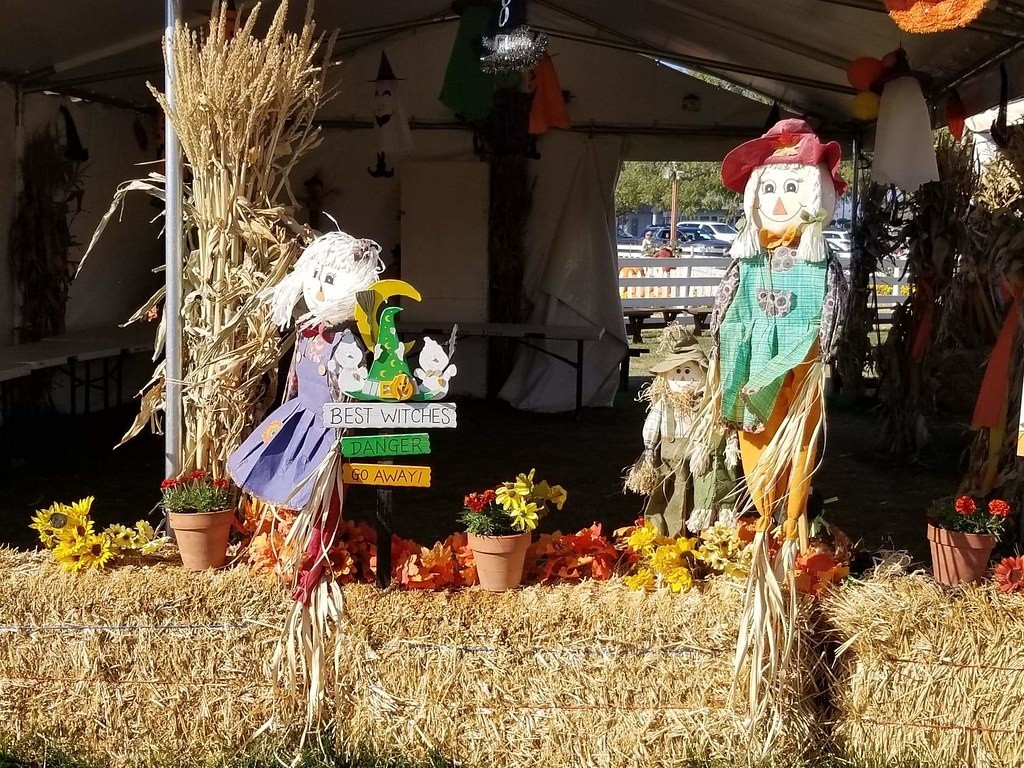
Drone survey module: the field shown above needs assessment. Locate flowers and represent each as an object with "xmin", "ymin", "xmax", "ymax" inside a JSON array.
[
  {"xmin": 928, "ymin": 495, "xmax": 1010, "ymax": 542},
  {"xmin": 993, "ymin": 555, "xmax": 1024, "ymax": 595},
  {"xmin": 28, "ymin": 495, "xmax": 172, "ymax": 576},
  {"xmin": 523, "ymin": 514, "xmax": 853, "ymax": 600},
  {"xmin": 453, "ymin": 467, "xmax": 566, "ymax": 536},
  {"xmin": 222, "ymin": 498, "xmax": 481, "ymax": 591},
  {"xmin": 149, "ymin": 468, "xmax": 228, "ymax": 516}
]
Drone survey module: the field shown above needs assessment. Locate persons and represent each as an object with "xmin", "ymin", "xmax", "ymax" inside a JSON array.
[
  {"xmin": 709, "ymin": 118, "xmax": 856, "ymax": 679},
  {"xmin": 641, "ymin": 231, "xmax": 678, "ymax": 271},
  {"xmin": 224, "ymin": 233, "xmax": 387, "ymax": 729},
  {"xmin": 623, "ymin": 323, "xmax": 737, "ymax": 539}
]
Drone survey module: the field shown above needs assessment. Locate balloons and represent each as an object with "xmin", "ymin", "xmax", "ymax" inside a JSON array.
[
  {"xmin": 853, "ymin": 91, "xmax": 880, "ymax": 120},
  {"xmin": 848, "ymin": 57, "xmax": 883, "ymax": 89}
]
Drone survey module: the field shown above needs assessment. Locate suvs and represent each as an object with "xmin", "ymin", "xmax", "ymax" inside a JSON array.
[
  {"xmin": 641, "ymin": 224, "xmax": 732, "ymax": 258},
  {"xmin": 666, "ymin": 222, "xmax": 740, "ymax": 248}
]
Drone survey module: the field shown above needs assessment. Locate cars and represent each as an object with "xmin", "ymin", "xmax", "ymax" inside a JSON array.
[{"xmin": 822, "ymin": 230, "xmax": 851, "ymax": 252}]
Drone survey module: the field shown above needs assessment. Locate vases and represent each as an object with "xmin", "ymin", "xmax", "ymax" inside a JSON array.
[
  {"xmin": 467, "ymin": 531, "xmax": 532, "ymax": 591},
  {"xmin": 925, "ymin": 521, "xmax": 998, "ymax": 587},
  {"xmin": 168, "ymin": 510, "xmax": 234, "ymax": 570}
]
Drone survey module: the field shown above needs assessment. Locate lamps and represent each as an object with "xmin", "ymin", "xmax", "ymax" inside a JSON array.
[
  {"xmin": 43, "ymin": 87, "xmax": 94, "ymax": 103},
  {"xmin": 870, "ymin": 43, "xmax": 941, "ymax": 194},
  {"xmin": 368, "ymin": 48, "xmax": 416, "ymax": 180}
]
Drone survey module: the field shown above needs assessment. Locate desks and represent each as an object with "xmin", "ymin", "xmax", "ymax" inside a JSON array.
[{"xmin": 0, "ymin": 321, "xmax": 606, "ymax": 430}]
